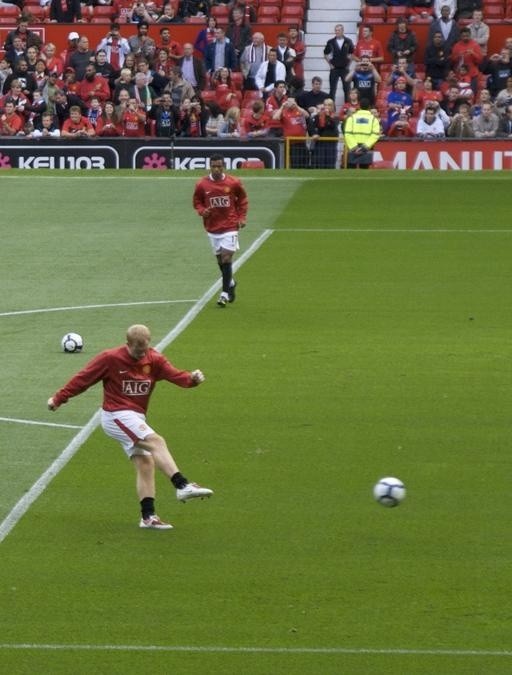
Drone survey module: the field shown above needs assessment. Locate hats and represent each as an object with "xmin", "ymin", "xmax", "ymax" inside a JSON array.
[
  {"xmin": 48, "ymin": 71, "xmax": 58, "ymax": 77},
  {"xmin": 65, "ymin": 67, "xmax": 75, "ymax": 74},
  {"xmin": 67, "ymin": 32, "xmax": 80, "ymax": 40}
]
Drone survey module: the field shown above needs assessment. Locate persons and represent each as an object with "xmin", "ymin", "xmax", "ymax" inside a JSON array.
[
  {"xmin": 192, "ymin": 153, "xmax": 248, "ymax": 306},
  {"xmin": 46, "ymin": 324, "xmax": 214, "ymax": 530}
]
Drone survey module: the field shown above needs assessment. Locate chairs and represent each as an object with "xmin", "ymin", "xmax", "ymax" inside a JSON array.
[{"xmin": 0, "ymin": 0, "xmax": 511, "ymax": 139}]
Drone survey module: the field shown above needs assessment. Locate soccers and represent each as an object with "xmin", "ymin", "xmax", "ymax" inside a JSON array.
[
  {"xmin": 374, "ymin": 478, "xmax": 406, "ymax": 507},
  {"xmin": 62, "ymin": 333, "xmax": 82, "ymax": 352}
]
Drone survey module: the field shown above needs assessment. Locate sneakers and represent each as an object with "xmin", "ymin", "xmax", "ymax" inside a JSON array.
[
  {"xmin": 176, "ymin": 481, "xmax": 214, "ymax": 504},
  {"xmin": 216, "ymin": 296, "xmax": 228, "ymax": 308},
  {"xmin": 139, "ymin": 514, "xmax": 174, "ymax": 530},
  {"xmin": 228, "ymin": 281, "xmax": 237, "ymax": 303}
]
